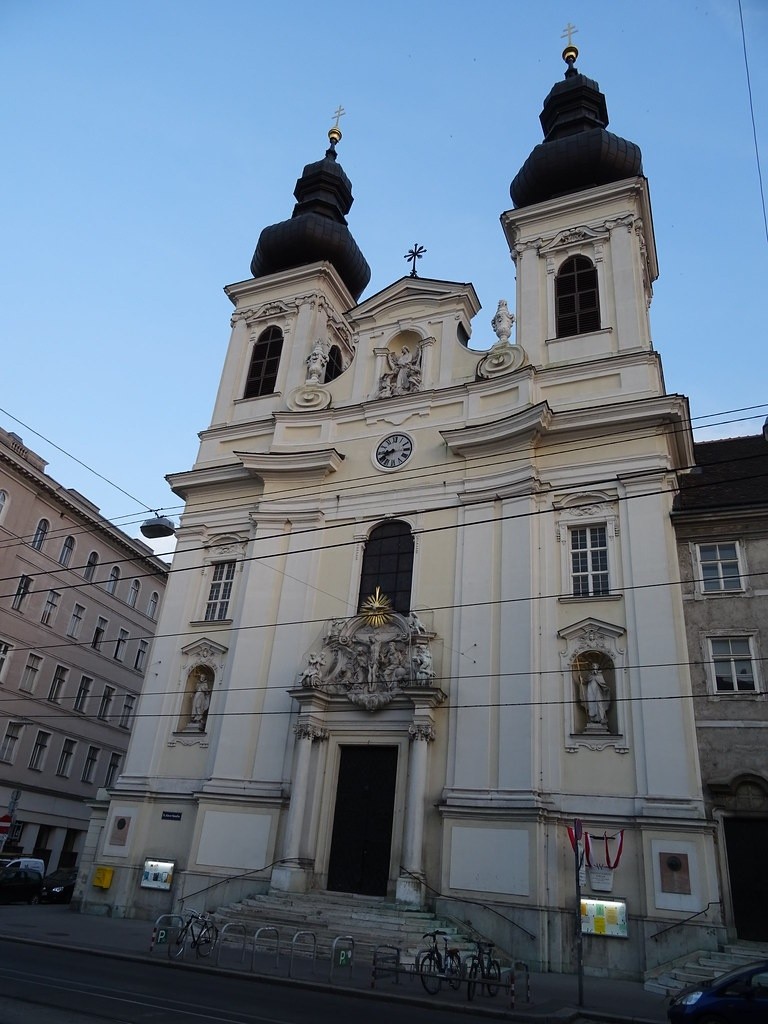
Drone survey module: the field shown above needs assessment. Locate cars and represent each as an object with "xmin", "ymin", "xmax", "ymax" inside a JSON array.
[
  {"xmin": 40, "ymin": 865, "xmax": 79, "ymax": 906},
  {"xmin": 0, "ymin": 866, "xmax": 44, "ymax": 906},
  {"xmin": 664, "ymin": 959, "xmax": 768, "ymax": 1023}
]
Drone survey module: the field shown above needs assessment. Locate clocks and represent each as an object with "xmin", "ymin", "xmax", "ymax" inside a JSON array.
[{"xmin": 372, "ymin": 431, "xmax": 415, "ymax": 472}]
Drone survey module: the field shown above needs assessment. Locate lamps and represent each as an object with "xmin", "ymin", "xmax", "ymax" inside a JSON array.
[{"xmin": 141, "ymin": 511, "xmax": 176, "ymax": 539}]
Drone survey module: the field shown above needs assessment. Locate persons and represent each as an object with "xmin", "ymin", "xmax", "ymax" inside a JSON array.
[
  {"xmin": 413, "ymin": 645, "xmax": 437, "ymax": 677},
  {"xmin": 325, "ymin": 619, "xmax": 344, "ymax": 644},
  {"xmin": 396, "ymin": 345, "xmax": 413, "ymax": 388},
  {"xmin": 412, "ymin": 612, "xmax": 427, "ymax": 635},
  {"xmin": 298, "ymin": 652, "xmax": 327, "ymax": 683},
  {"xmin": 579, "ymin": 663, "xmax": 610, "ymax": 724},
  {"xmin": 354, "ymin": 631, "xmax": 403, "ymax": 692},
  {"xmin": 191, "ymin": 674, "xmax": 209, "ymax": 721}
]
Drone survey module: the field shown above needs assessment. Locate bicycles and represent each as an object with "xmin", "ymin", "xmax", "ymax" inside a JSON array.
[
  {"xmin": 460, "ymin": 935, "xmax": 503, "ymax": 1001},
  {"xmin": 420, "ymin": 928, "xmax": 464, "ymax": 995},
  {"xmin": 168, "ymin": 908, "xmax": 219, "ymax": 961}
]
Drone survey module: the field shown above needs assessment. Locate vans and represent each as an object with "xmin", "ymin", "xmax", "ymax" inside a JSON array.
[{"xmin": 0, "ymin": 857, "xmax": 45, "ymax": 881}]
